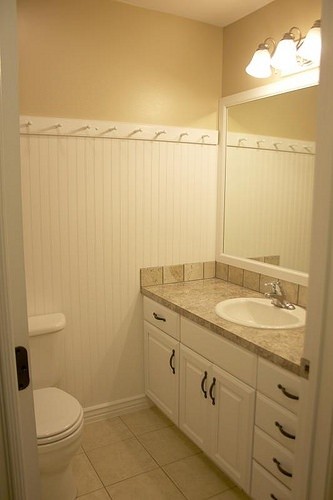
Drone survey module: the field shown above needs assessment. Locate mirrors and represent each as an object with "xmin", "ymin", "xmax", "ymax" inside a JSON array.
[{"xmin": 215, "ymin": 67, "xmax": 320, "ymax": 287}]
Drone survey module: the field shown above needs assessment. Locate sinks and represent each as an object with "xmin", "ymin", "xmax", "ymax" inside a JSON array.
[{"xmin": 222, "ymin": 300, "xmax": 299, "ymax": 326}]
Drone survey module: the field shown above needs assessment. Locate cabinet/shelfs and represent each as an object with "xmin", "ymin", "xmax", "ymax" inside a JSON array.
[
  {"xmin": 178, "ymin": 316, "xmax": 259, "ymax": 496},
  {"xmin": 142, "ymin": 295, "xmax": 181, "ymax": 424},
  {"xmin": 249, "ymin": 355, "xmax": 308, "ymax": 499}
]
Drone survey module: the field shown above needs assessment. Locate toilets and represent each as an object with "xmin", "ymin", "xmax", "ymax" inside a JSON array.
[{"xmin": 33, "ymin": 388, "xmax": 84, "ymax": 500}]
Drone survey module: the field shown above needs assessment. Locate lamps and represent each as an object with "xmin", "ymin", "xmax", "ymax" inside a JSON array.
[
  {"xmin": 297, "ymin": 19, "xmax": 321, "ymax": 64},
  {"xmin": 245, "ymin": 36, "xmax": 276, "ymax": 78},
  {"xmin": 270, "ymin": 26, "xmax": 301, "ymax": 72}
]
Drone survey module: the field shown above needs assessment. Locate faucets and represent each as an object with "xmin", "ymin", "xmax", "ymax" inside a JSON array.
[{"xmin": 265, "ymin": 293, "xmax": 294, "ymax": 309}]
